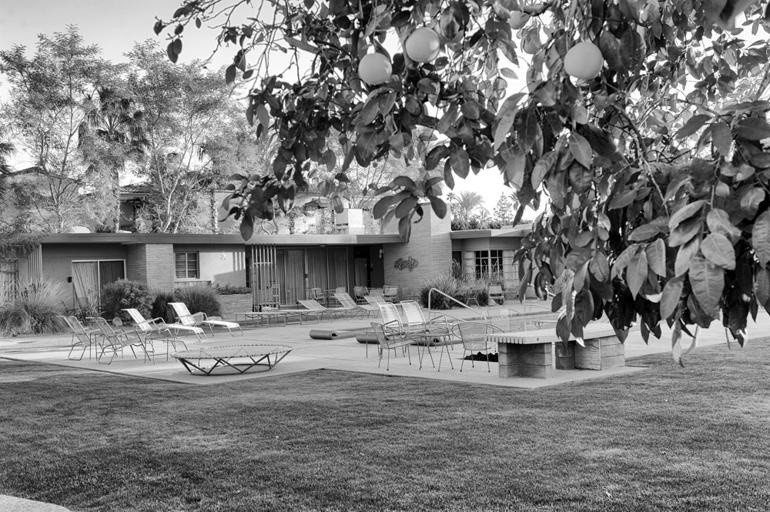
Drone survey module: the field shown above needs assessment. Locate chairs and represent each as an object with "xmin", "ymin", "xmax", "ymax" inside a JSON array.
[
  {"xmin": 370, "ymin": 300, "xmax": 504, "ymax": 372},
  {"xmin": 297, "ymin": 286, "xmax": 398, "ymax": 320},
  {"xmin": 56, "ymin": 300, "xmax": 245, "ymax": 364}
]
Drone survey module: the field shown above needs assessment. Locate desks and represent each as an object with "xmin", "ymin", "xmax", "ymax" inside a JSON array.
[{"xmin": 234, "ymin": 312, "xmax": 288, "ymax": 327}]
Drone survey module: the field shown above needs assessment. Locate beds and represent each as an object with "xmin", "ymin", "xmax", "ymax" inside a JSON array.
[{"xmin": 309, "ymin": 326, "xmax": 380, "ymax": 343}]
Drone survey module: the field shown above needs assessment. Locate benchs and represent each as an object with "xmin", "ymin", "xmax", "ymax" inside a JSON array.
[{"xmin": 486, "ymin": 318, "xmax": 629, "ymax": 379}]
